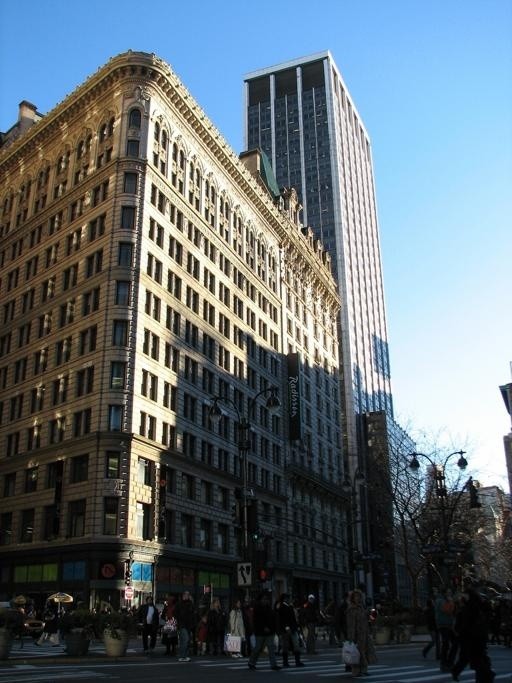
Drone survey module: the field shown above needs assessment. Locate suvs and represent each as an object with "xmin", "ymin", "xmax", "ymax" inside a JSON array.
[{"xmin": 1, "ymin": 606, "xmax": 45, "ymax": 639}]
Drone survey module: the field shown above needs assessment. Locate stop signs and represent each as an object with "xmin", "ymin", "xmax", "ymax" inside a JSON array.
[{"xmin": 124, "ymin": 586, "xmax": 134, "ymax": 600}]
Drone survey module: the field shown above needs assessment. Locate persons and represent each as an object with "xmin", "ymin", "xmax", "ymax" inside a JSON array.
[
  {"xmin": 93, "ymin": 592, "xmax": 307, "ymax": 671},
  {"xmin": 16, "ymin": 605, "xmax": 67, "ymax": 649},
  {"xmin": 298, "ymin": 589, "xmax": 512, "ymax": 683}
]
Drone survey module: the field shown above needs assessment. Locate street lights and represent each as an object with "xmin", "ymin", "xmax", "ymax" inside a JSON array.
[
  {"xmin": 208, "ymin": 388, "xmax": 282, "ymax": 598},
  {"xmin": 409, "ymin": 448, "xmax": 468, "ymax": 555},
  {"xmin": 340, "ymin": 463, "xmax": 367, "ymax": 588}
]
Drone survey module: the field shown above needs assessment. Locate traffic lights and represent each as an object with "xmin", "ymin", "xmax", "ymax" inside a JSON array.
[
  {"xmin": 258, "ymin": 566, "xmax": 273, "ymax": 581},
  {"xmin": 125, "ymin": 570, "xmax": 133, "ymax": 584}
]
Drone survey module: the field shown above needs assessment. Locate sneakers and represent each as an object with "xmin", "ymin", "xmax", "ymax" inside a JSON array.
[
  {"xmin": 177, "ymin": 657, "xmax": 191, "ymax": 661},
  {"xmin": 247, "ymin": 664, "xmax": 256, "ymax": 670},
  {"xmin": 271, "ymin": 661, "xmax": 304, "ymax": 670},
  {"xmin": 422, "ymin": 651, "xmax": 427, "ymax": 658},
  {"xmin": 451, "ymin": 672, "xmax": 460, "ymax": 681},
  {"xmin": 232, "ymin": 653, "xmax": 242, "ymax": 658},
  {"xmin": 345, "ymin": 666, "xmax": 370, "ymax": 677}
]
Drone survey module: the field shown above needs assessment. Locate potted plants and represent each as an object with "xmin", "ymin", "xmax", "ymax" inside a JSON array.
[
  {"xmin": 0, "ymin": 607, "xmax": 26, "ymax": 659},
  {"xmin": 55, "ymin": 607, "xmax": 137, "ymax": 656}
]
